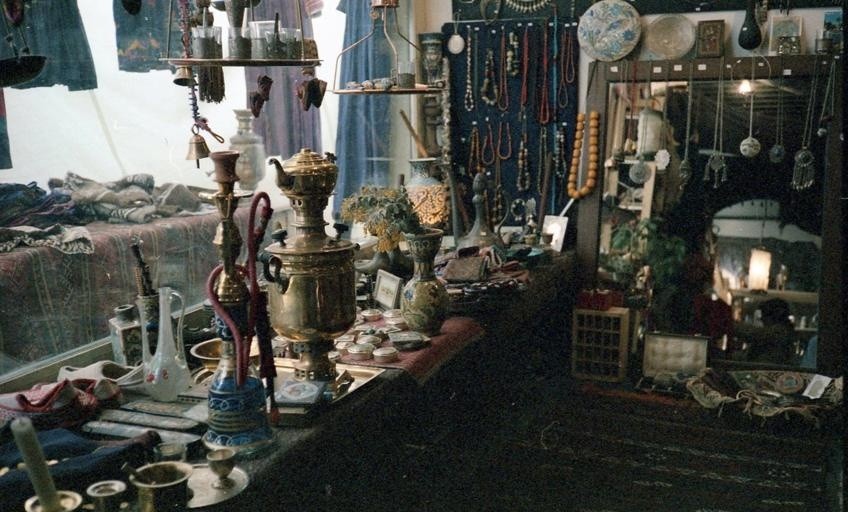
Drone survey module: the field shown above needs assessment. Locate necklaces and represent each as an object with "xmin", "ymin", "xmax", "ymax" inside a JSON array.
[
  {"xmin": 816, "ymin": 59, "xmax": 836, "ymax": 138},
  {"xmin": 604, "ymin": 63, "xmax": 624, "ymax": 169},
  {"xmin": 481, "ymin": 123, "xmax": 495, "ymax": 166},
  {"xmin": 491, "ymin": 159, "xmax": 503, "ymax": 226},
  {"xmin": 655, "ymin": 60, "xmax": 671, "ymax": 171},
  {"xmin": 497, "ymin": 120, "xmax": 512, "ymax": 160},
  {"xmin": 516, "ymin": 119, "xmax": 531, "ymax": 192},
  {"xmin": 740, "ymin": 56, "xmax": 761, "ymax": 157},
  {"xmin": 536, "ymin": 126, "xmax": 548, "ymax": 195},
  {"xmin": 505, "ymin": 0, "xmax": 552, "ymax": 13},
  {"xmin": 791, "ymin": 54, "xmax": 823, "ymax": 192},
  {"xmin": 555, "ymin": 126, "xmax": 566, "ymax": 179},
  {"xmin": 567, "ymin": 110, "xmax": 599, "ymax": 200},
  {"xmin": 539, "ymin": 16, "xmax": 550, "ymax": 125},
  {"xmin": 770, "ymin": 54, "xmax": 786, "ymax": 164},
  {"xmin": 520, "ymin": 25, "xmax": 529, "ymax": 105},
  {"xmin": 506, "ymin": 25, "xmax": 520, "ymax": 79},
  {"xmin": 558, "ymin": 22, "xmax": 569, "ymax": 109},
  {"xmin": 498, "ymin": 30, "xmax": 508, "ymax": 111},
  {"xmin": 480, "ymin": 49, "xmax": 499, "ymax": 106},
  {"xmin": 479, "ymin": 0, "xmax": 500, "ymax": 26},
  {"xmin": 464, "ymin": 28, "xmax": 474, "ymax": 112},
  {"xmin": 565, "ymin": 24, "xmax": 575, "ymax": 83},
  {"xmin": 629, "ymin": 59, "xmax": 654, "ymax": 184},
  {"xmin": 624, "ymin": 60, "xmax": 638, "ymax": 155},
  {"xmin": 678, "ymin": 58, "xmax": 693, "ymax": 191},
  {"xmin": 448, "ymin": 12, "xmax": 464, "ymax": 58},
  {"xmin": 468, "ymin": 126, "xmax": 480, "ymax": 179},
  {"xmin": 703, "ymin": 56, "xmax": 727, "ymax": 188}
]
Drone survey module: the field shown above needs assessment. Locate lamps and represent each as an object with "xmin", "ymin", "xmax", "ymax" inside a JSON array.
[{"xmin": 745, "ymin": 199, "xmax": 771, "ymax": 292}]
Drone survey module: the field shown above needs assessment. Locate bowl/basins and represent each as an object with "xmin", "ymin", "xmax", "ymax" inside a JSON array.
[{"xmin": 190, "ymin": 335, "xmax": 276, "ymax": 376}]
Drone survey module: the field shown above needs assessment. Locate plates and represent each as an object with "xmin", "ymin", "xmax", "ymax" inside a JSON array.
[
  {"xmin": 644, "ymin": 14, "xmax": 696, "ymax": 61},
  {"xmin": 189, "ymin": 357, "xmax": 385, "ymax": 406},
  {"xmin": 188, "ymin": 463, "xmax": 250, "ymax": 509}
]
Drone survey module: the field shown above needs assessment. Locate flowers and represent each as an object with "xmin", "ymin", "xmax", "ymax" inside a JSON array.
[{"xmin": 342, "ymin": 183, "xmax": 414, "ymax": 249}]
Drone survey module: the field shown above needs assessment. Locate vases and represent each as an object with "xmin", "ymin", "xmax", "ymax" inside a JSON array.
[
  {"xmin": 367, "ymin": 246, "xmax": 406, "ymax": 285},
  {"xmin": 112, "ymin": 304, "xmax": 143, "ymax": 368},
  {"xmin": 408, "ymin": 156, "xmax": 446, "ymax": 230},
  {"xmin": 405, "ymin": 231, "xmax": 447, "ymax": 339}
]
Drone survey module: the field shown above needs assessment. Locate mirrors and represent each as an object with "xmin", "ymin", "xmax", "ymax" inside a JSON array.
[{"xmin": 575, "ymin": 56, "xmax": 844, "ymax": 377}]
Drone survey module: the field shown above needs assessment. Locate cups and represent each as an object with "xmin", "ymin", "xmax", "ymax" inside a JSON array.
[
  {"xmin": 524, "ymin": 234, "xmax": 537, "ymax": 246},
  {"xmin": 207, "ymin": 449, "xmax": 236, "ymax": 490},
  {"xmin": 542, "ymin": 234, "xmax": 553, "ymax": 249}
]
